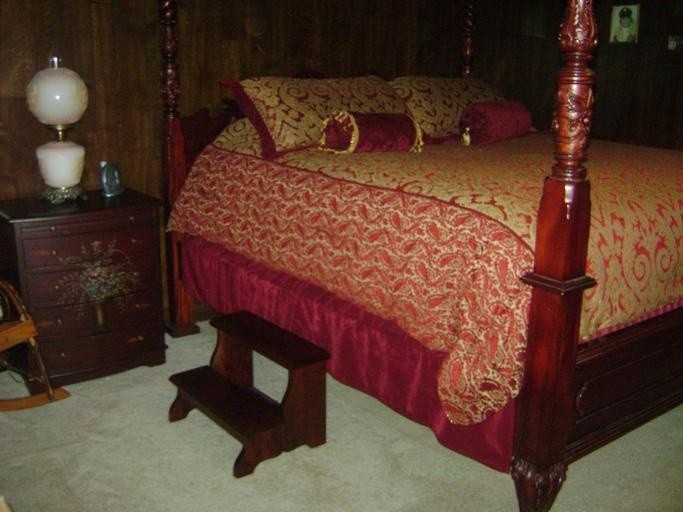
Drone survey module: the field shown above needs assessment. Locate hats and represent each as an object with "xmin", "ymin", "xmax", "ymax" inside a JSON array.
[{"xmin": 619, "ymin": 7, "xmax": 631, "ymax": 17}]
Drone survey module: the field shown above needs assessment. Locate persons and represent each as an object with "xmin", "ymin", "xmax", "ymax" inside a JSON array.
[{"xmin": 610, "ymin": 6, "xmax": 637, "ymax": 42}]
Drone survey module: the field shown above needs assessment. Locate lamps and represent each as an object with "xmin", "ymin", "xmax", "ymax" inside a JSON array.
[{"xmin": 23, "ymin": 55, "xmax": 91, "ymax": 207}]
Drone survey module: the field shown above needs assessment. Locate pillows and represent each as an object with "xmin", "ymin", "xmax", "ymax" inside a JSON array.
[
  {"xmin": 385, "ymin": 76, "xmax": 505, "ymax": 147},
  {"xmin": 315, "ymin": 104, "xmax": 424, "ymax": 157},
  {"xmin": 458, "ymin": 99, "xmax": 532, "ymax": 146},
  {"xmin": 213, "ymin": 68, "xmax": 424, "ymax": 160}
]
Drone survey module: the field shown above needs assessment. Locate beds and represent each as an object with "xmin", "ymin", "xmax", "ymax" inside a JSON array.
[{"xmin": 156, "ymin": 1, "xmax": 681, "ymax": 512}]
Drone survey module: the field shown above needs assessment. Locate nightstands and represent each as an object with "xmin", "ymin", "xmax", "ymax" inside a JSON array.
[{"xmin": 0, "ymin": 186, "xmax": 167, "ymax": 397}]
